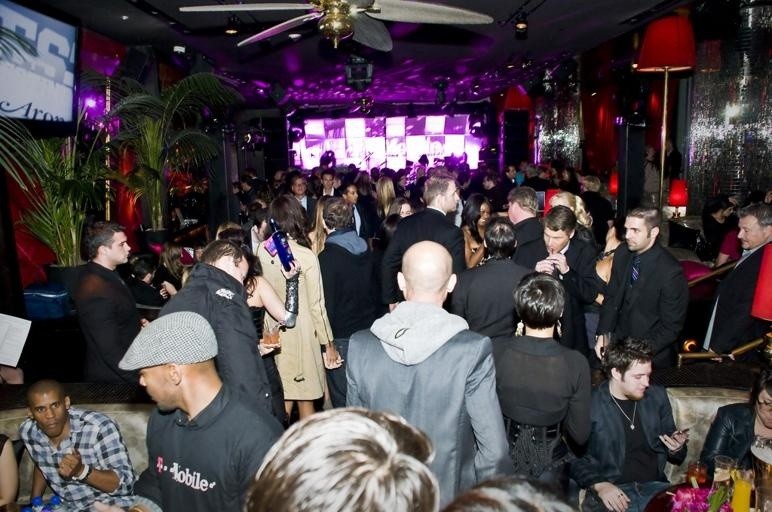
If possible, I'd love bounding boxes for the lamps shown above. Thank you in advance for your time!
[750,243,772,372]
[636,15,695,213]
[310,7,354,47]
[667,179,688,217]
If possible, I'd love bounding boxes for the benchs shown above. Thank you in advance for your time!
[5,382,148,407]
[0,403,156,512]
[579,386,749,512]
[136,301,161,321]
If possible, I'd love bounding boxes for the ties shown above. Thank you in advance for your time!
[630,257,640,284]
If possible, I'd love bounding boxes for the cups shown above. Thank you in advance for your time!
[728,468,752,511]
[685,458,708,486]
[261,328,281,346]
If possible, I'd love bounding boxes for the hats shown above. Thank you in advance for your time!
[117,310,218,373]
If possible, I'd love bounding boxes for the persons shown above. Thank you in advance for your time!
[0,141,772,512]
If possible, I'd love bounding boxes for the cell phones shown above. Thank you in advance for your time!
[665,428,690,441]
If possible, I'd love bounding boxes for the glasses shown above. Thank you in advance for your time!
[759,400,772,412]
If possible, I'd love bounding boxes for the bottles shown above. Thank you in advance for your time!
[711,455,736,501]
[22,494,67,512]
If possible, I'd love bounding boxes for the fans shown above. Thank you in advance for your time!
[179,0,496,55]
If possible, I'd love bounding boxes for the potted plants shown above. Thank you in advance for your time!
[0,80,159,291]
[75,57,242,253]
